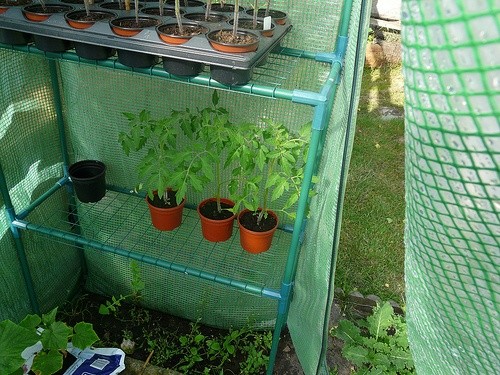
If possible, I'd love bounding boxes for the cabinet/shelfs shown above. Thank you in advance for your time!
[0,0,360,375]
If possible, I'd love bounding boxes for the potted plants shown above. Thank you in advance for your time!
[0,0,333,256]
